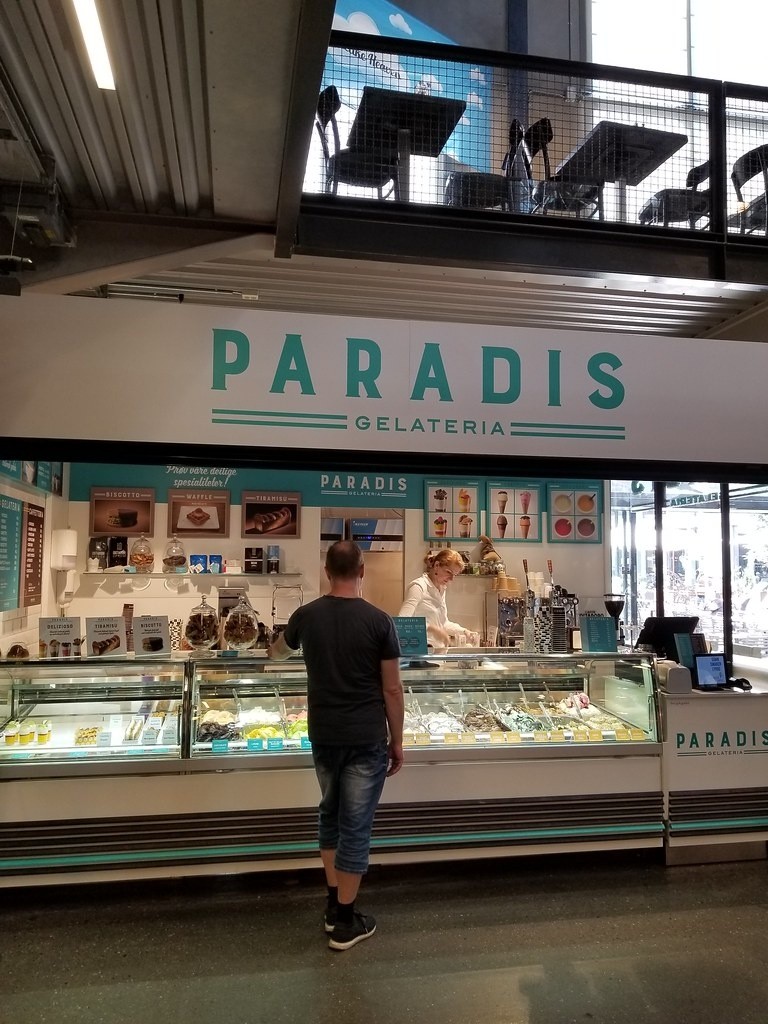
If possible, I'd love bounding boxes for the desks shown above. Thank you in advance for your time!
[556,121,688,223]
[345,86,467,202]
[727,192,765,230]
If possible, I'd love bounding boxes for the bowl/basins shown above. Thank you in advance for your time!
[554,494,573,512]
[576,494,595,514]
[553,518,573,538]
[576,517,596,538]
[522,605,568,652]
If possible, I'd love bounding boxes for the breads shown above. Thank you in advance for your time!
[187,508,210,523]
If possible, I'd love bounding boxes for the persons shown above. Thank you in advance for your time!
[399,549,478,656]
[266,540,404,948]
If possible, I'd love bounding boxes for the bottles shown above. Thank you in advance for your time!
[129,531,155,573]
[458,551,506,575]
[272,583,304,633]
[185,595,259,657]
[424,540,452,573]
[162,533,187,573]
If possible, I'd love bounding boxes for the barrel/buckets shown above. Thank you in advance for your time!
[189,554,223,573]
[266,545,280,573]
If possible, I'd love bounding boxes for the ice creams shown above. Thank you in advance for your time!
[520,516,532,539]
[520,491,531,514]
[497,515,508,538]
[497,490,508,513]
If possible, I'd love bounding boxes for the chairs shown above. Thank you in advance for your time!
[444,117,601,217]
[639,160,711,232]
[316,85,399,201]
[732,144,768,236]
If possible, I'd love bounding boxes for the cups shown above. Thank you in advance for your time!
[5,729,52,745]
[450,632,481,647]
[74,645,81,656]
[49,645,59,657]
[434,496,471,537]
[493,572,520,591]
[528,572,555,597]
[40,645,47,657]
[423,645,448,654]
[62,645,72,657]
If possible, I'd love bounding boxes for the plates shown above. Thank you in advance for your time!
[176,505,219,529]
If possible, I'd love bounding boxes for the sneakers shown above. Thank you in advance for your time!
[328,908,376,950]
[324,909,336,931]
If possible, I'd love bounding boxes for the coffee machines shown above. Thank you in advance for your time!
[602,593,625,645]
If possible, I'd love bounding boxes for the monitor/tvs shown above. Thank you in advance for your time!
[674,634,728,691]
[633,617,700,664]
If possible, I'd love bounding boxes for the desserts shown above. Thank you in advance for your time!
[75,726,102,745]
[255,507,291,533]
[106,508,138,527]
[4,719,53,744]
[554,495,595,537]
